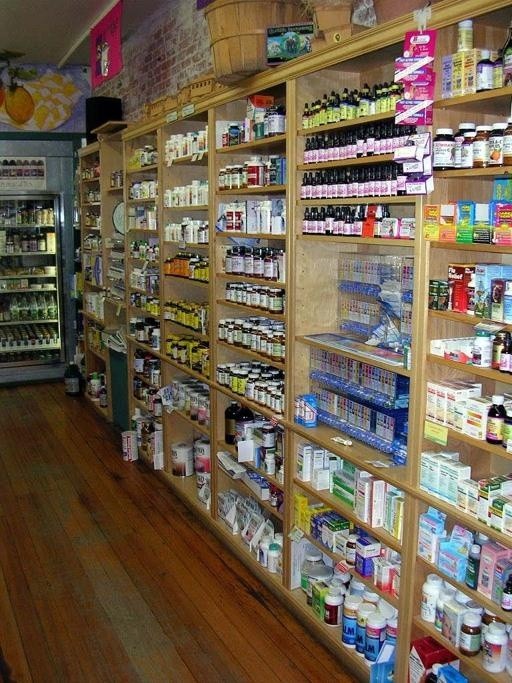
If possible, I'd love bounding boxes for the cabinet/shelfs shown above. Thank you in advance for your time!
[123,117,161,478]
[161,106,211,530]
[101,121,125,310]
[78,140,119,422]
[394,3,509,683]
[285,22,421,683]
[208,69,290,597]
[0,193,67,366]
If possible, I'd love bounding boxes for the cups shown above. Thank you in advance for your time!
[30,283,55,289]
[44,266,56,275]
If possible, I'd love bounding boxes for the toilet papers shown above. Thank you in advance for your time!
[195,438,212,473]
[197,471,212,502]
[170,442,195,478]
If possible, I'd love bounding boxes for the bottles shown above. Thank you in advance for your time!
[79,167,101,229]
[254,103,286,140]
[417,572,512,683]
[216,242,286,508]
[87,371,107,408]
[299,79,417,239]
[346,536,360,565]
[129,126,210,429]
[0,204,59,254]
[2,350,59,366]
[300,546,397,665]
[471,330,512,375]
[256,199,271,233]
[222,122,244,147]
[454,19,473,52]
[475,21,512,91]
[463,533,490,589]
[432,115,512,171]
[131,407,144,446]
[0,293,58,321]
[64,360,84,397]
[486,392,511,448]
[219,152,280,189]
[258,532,282,575]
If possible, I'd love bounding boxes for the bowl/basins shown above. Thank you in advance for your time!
[202,0,308,86]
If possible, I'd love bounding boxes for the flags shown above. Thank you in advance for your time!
[88,0,125,90]
